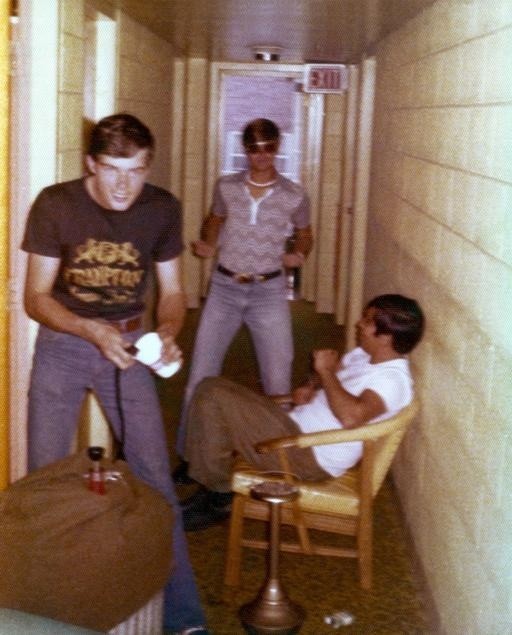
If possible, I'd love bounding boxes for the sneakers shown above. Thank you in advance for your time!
[171,459,198,486]
[180,486,235,532]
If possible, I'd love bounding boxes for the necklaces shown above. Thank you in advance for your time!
[244,169,278,188]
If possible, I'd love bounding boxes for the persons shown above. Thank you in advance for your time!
[18,113,211,635]
[179,294,426,537]
[167,117,314,487]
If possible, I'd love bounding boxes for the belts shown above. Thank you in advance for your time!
[216,264,282,285]
[111,316,142,335]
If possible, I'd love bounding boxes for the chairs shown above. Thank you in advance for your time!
[219,382,421,590]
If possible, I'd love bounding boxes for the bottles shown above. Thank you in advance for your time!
[85,444,108,498]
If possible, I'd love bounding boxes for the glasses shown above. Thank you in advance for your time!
[246,143,277,154]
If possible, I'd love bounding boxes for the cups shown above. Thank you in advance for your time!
[130,330,181,379]
